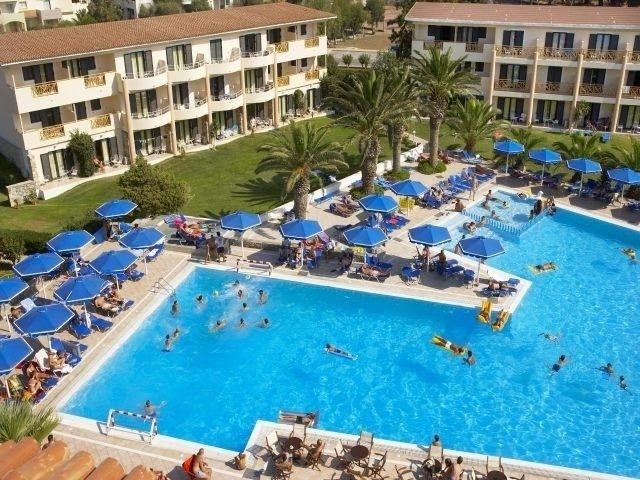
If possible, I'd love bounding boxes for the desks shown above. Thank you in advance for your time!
[350,444,368,462]
[485,470,505,480]
[421,459,444,475]
[285,437,302,455]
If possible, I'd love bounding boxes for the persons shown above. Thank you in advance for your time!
[358,266,390,278]
[429,186,444,201]
[343,196,360,208]
[468,222,477,232]
[462,351,475,366]
[586,116,597,131]
[282,236,335,268]
[479,307,490,323]
[599,362,615,375]
[42,434,53,450]
[430,336,464,355]
[280,413,315,428]
[8,220,138,395]
[614,246,635,253]
[93,155,103,170]
[455,199,465,212]
[160,223,270,353]
[491,210,497,218]
[537,333,561,346]
[137,400,157,418]
[433,249,446,263]
[192,435,463,480]
[326,343,348,356]
[483,190,492,209]
[469,175,479,201]
[618,376,628,389]
[545,355,568,379]
[419,246,430,261]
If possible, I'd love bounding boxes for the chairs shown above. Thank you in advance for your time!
[427,445,446,463]
[22,300,42,314]
[344,463,366,478]
[288,426,308,446]
[267,432,282,454]
[484,456,506,471]
[275,234,520,298]
[368,453,391,478]
[471,467,484,480]
[67,319,89,338]
[329,147,497,234]
[334,438,352,462]
[106,289,137,306]
[356,431,375,455]
[91,314,113,335]
[509,166,640,213]
[272,463,297,479]
[62,261,83,279]
[37,350,68,371]
[306,445,326,470]
[5,379,36,401]
[126,268,144,281]
[508,112,640,134]
[176,233,197,250]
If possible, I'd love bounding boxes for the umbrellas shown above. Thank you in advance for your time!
[219,180,506,283]
[493,139,640,208]
[0,199,166,399]
